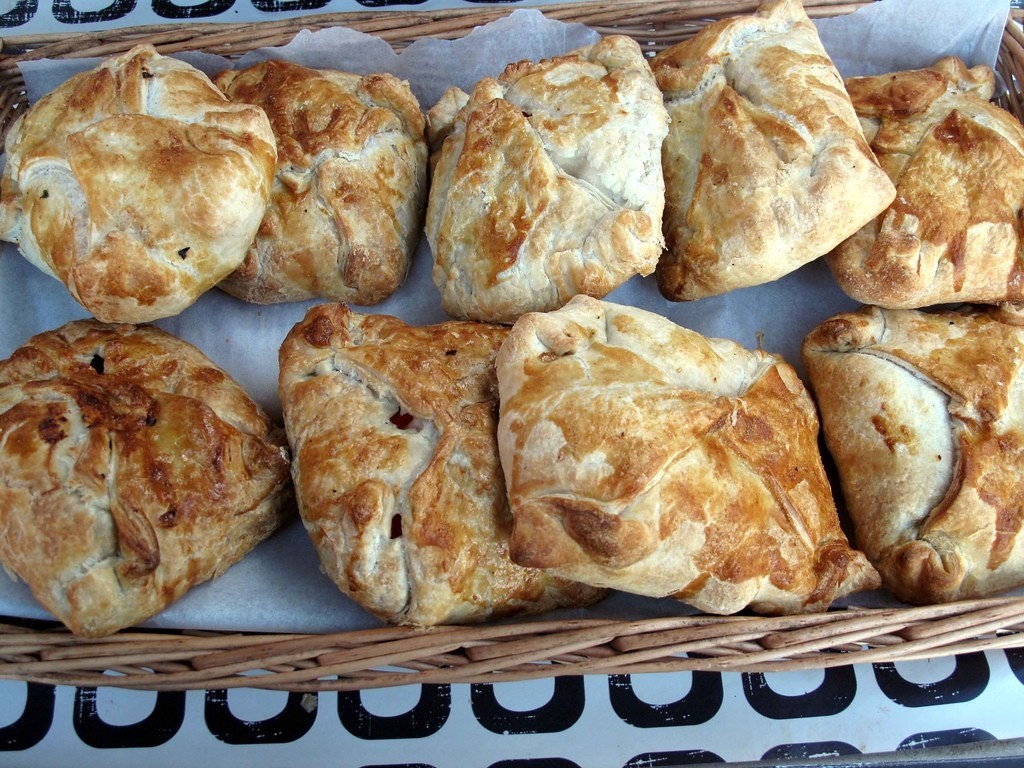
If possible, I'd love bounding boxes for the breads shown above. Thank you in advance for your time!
[1,0,1024,638]
